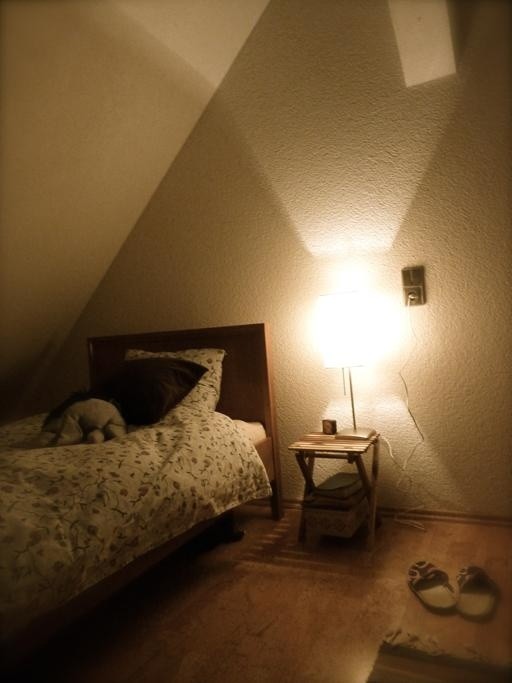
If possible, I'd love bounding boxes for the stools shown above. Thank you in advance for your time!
[288,431,380,552]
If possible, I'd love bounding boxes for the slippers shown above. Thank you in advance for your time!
[407,560,499,618]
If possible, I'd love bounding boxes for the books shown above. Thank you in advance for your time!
[315,471,363,499]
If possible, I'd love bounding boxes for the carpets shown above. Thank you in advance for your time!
[366,627,512,683]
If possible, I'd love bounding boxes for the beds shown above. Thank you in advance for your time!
[0,322,284,678]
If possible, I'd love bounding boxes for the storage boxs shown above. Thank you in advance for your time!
[303,496,369,538]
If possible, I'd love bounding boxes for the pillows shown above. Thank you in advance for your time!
[119,347,228,411]
[40,357,209,431]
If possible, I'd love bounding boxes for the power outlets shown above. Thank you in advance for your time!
[401,266,425,307]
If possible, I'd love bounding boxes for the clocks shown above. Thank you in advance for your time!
[322,419,336,434]
